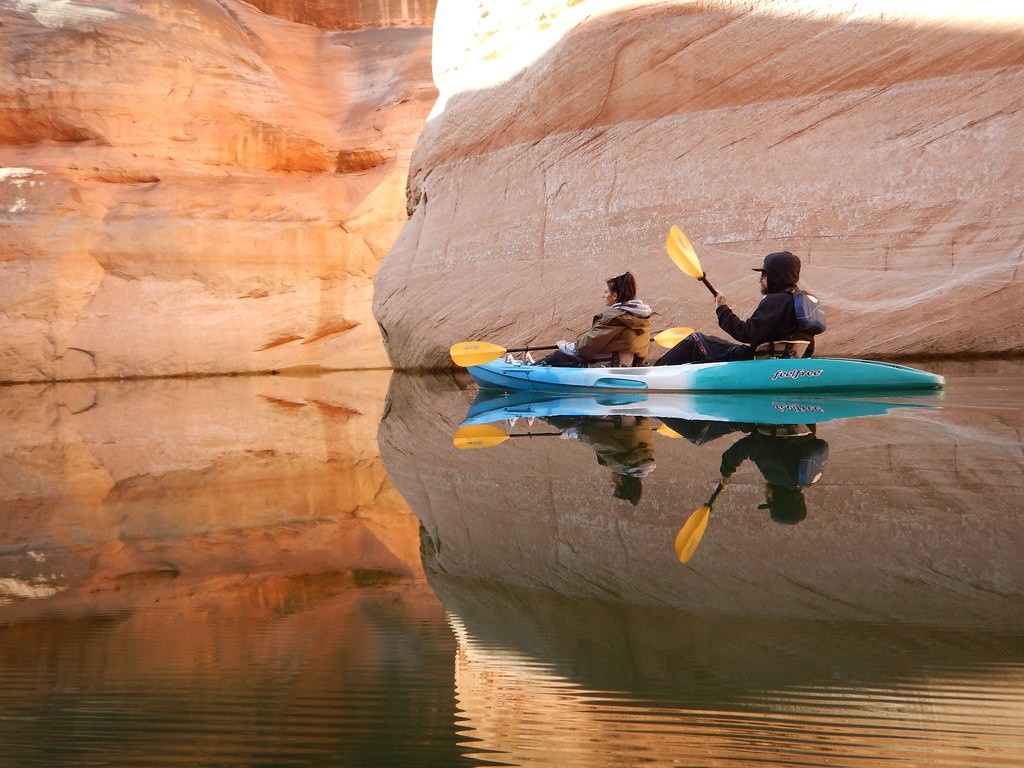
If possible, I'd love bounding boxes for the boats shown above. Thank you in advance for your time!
[466,341,948,389]
[457,389,945,437]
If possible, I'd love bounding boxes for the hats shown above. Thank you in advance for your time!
[752,268,764,271]
[758,504,770,510]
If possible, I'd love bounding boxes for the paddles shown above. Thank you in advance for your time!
[450,326,697,369]
[666,225,717,297]
[675,483,724,565]
[452,419,682,449]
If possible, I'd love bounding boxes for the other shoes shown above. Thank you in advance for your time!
[506,417,520,427]
[523,351,535,367]
[526,416,535,427]
[504,353,517,365]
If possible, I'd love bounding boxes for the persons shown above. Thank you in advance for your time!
[508,416,655,506]
[653,251,827,367]
[657,418,829,525]
[505,271,651,367]
